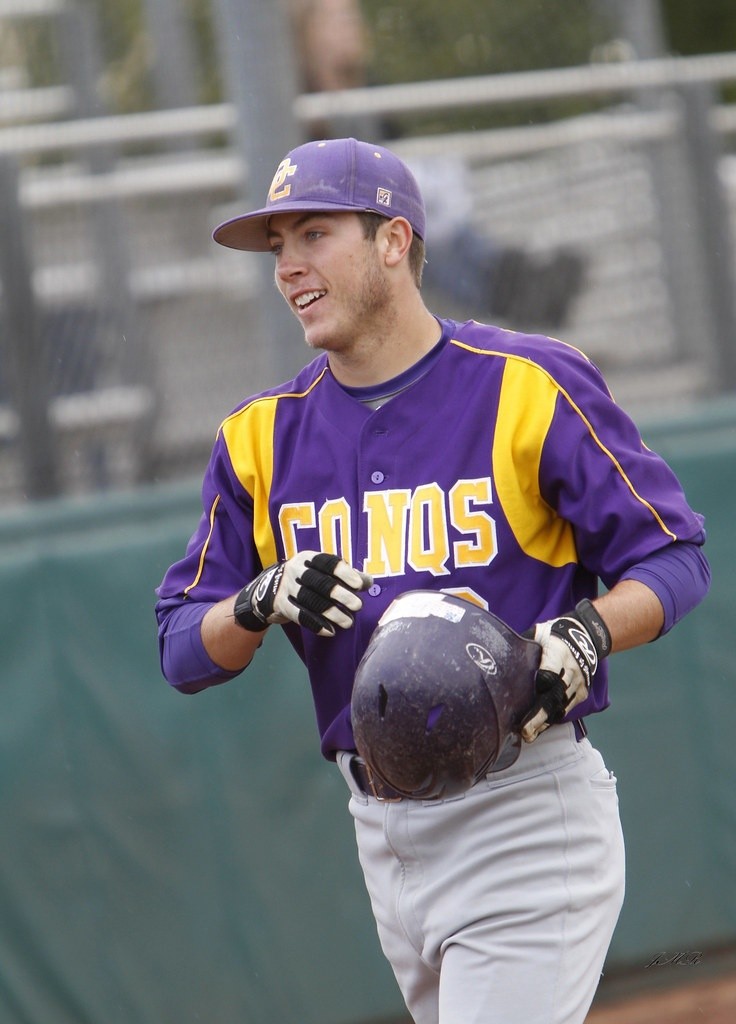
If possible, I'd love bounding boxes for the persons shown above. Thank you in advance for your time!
[158,135,710,1023]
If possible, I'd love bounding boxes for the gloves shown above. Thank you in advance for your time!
[512,600,616,749]
[231,559,368,643]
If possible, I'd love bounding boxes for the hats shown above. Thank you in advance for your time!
[212,151,431,249]
